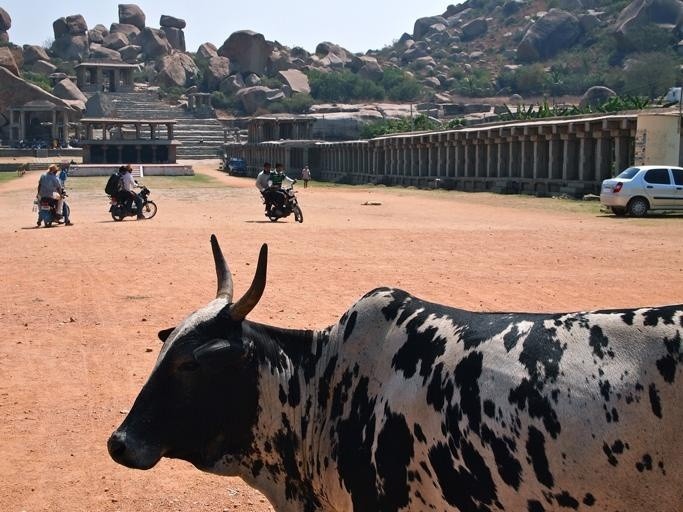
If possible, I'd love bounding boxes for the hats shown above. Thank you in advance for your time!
[48,164,60,173]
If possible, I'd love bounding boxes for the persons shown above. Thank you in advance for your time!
[111,166,134,214]
[255,162,275,215]
[36,175,74,227]
[267,164,298,216]
[302,165,311,188]
[36,164,66,221]
[123,164,146,220]
[58,169,66,187]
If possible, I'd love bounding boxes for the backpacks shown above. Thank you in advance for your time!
[104,172,122,195]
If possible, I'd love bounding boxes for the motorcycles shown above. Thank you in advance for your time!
[108,181,157,220]
[33,190,71,227]
[262,180,304,222]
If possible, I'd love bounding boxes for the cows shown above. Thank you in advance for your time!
[106,233,683,512]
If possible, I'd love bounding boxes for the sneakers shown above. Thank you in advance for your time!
[65,222,73,225]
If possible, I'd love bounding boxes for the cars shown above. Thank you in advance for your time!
[228,157,247,176]
[598,165,683,216]
